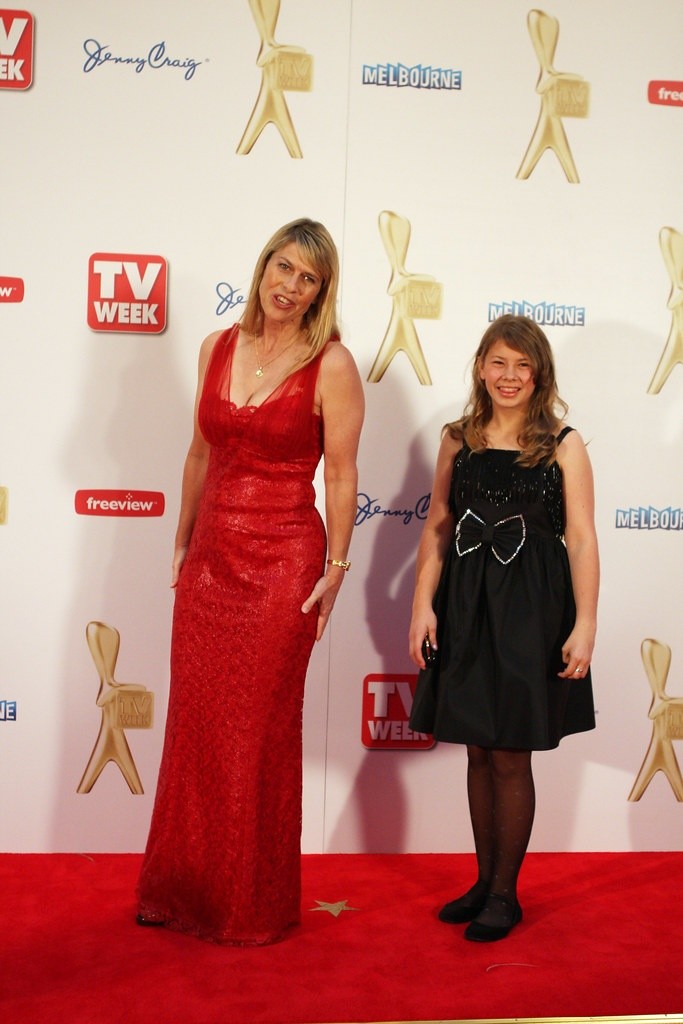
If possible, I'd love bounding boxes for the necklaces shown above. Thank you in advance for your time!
[253,332,298,378]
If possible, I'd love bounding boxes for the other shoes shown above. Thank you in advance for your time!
[134,913,165,926]
[439,884,484,923]
[465,891,522,943]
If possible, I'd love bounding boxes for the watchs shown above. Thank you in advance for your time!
[327,559,352,571]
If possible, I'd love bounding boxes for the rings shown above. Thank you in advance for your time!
[575,667,583,674]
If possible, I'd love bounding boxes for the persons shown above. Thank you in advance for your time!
[409,315,598,941]
[135,218,365,947]
